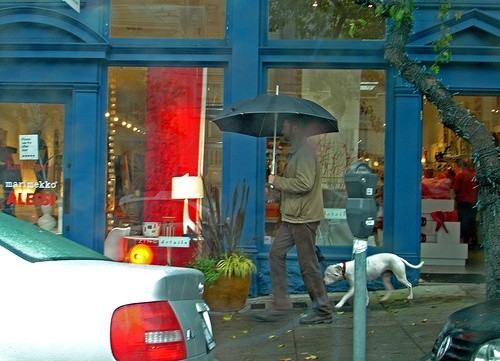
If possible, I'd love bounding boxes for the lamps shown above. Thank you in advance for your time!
[171,176,204,235]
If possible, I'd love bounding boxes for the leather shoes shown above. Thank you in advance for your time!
[252,311,292,323]
[298,315,333,327]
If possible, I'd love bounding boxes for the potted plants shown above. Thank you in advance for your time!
[182,177,257,312]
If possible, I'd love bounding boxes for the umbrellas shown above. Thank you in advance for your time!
[210,84,339,188]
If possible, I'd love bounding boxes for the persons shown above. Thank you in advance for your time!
[450,158,481,249]
[254,115,332,324]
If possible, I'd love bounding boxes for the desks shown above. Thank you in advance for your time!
[123,235,205,266]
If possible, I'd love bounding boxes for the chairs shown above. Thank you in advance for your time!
[104,226,131,261]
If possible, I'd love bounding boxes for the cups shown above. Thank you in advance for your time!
[162,216,176,238]
[142,221,161,238]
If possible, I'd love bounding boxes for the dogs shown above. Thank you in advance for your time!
[322,251,425,308]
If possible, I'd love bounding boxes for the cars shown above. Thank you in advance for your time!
[423,298,500,361]
[0,210,217,361]
[119,189,212,220]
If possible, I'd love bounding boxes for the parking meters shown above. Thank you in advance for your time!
[344,161,379,360]
